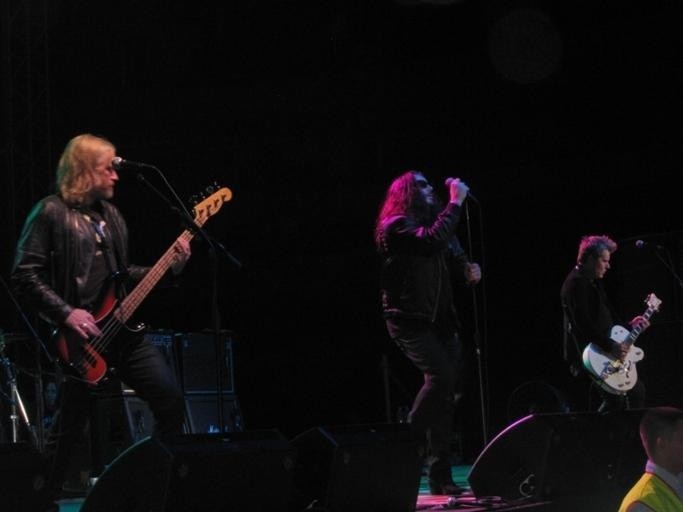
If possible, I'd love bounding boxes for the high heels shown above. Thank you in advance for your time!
[426,477,468,495]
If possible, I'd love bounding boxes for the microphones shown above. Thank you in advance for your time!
[635,239,661,251]
[110,156,157,174]
[445,178,479,205]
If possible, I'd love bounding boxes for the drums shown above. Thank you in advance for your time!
[507,379,571,435]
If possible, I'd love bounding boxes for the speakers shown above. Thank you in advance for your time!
[79,434,265,512]
[468,409,667,500]
[1,441,54,511]
[268,423,421,511]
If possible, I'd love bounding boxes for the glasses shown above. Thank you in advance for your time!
[412,179,431,189]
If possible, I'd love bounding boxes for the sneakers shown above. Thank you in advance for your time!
[62,470,100,492]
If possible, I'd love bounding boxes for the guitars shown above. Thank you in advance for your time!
[49,179,232,395]
[582,293,663,396]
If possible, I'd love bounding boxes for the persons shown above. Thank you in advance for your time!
[618,406,683,512]
[560,234,650,413]
[9,133,190,472]
[31,380,60,428]
[374,169,482,496]
